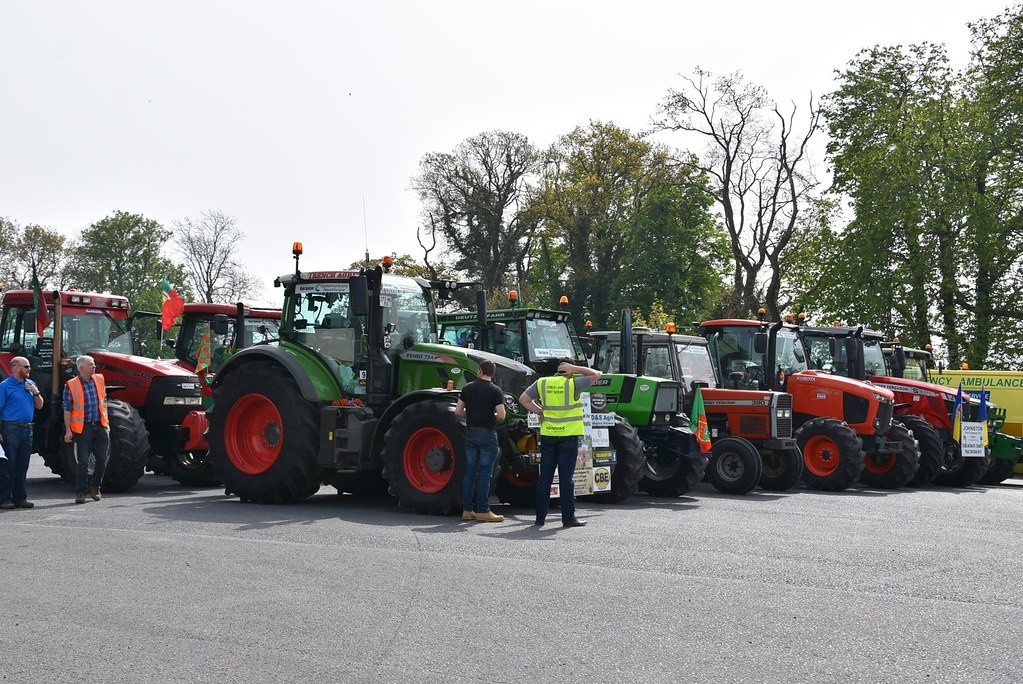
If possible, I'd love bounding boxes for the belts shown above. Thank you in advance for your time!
[471,427,493,432]
[1,420,33,427]
[84,421,101,425]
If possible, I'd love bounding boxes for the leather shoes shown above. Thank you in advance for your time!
[535,518,545,525]
[563,520,586,527]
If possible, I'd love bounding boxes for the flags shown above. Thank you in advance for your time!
[33,262,51,339]
[689,387,713,454]
[194,324,211,374]
[978,389,988,446]
[951,385,961,444]
[162,278,184,332]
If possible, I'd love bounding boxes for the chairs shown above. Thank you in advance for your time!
[33,337,67,367]
[321,313,352,342]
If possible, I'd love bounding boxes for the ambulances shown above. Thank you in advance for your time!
[841,345,1023,486]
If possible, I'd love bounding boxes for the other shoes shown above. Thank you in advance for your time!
[12,499,34,508]
[0,498,15,509]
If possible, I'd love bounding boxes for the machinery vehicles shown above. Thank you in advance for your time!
[582,318,804,495]
[693,313,921,494]
[331,308,607,360]
[784,314,1008,489]
[203,240,552,518]
[408,290,710,505]
[158,293,311,418]
[0,288,224,495]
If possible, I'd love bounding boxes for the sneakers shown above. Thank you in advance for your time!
[75,490,86,502]
[475,510,504,521]
[462,511,477,519]
[89,485,101,500]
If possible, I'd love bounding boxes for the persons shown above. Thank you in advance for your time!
[0,356,44,509]
[455,360,506,522]
[519,357,601,527]
[62,355,112,504]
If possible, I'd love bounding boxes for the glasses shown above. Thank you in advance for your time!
[13,365,32,369]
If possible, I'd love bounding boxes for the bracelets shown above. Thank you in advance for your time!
[33,392,40,396]
